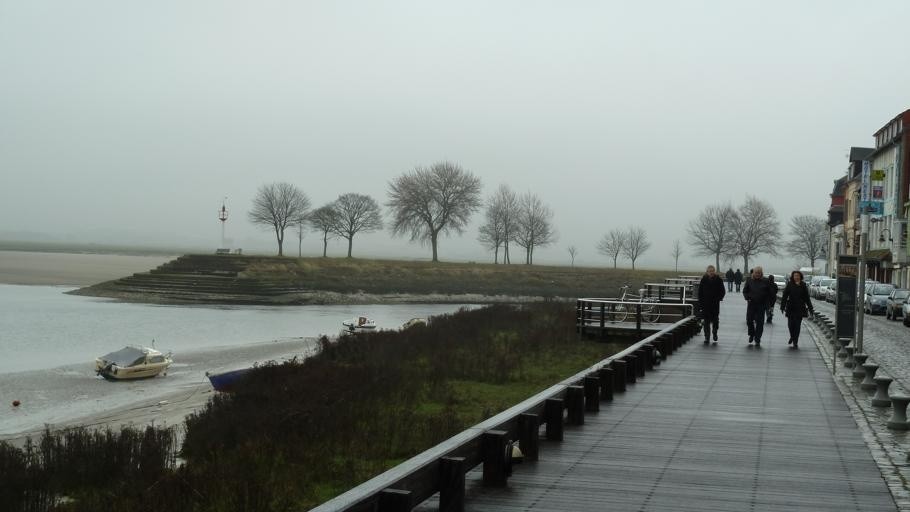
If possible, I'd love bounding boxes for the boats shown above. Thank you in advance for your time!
[399,317,427,331]
[343,316,377,328]
[94,343,174,381]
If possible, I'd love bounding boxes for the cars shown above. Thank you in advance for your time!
[802,275,910,326]
[773,275,786,290]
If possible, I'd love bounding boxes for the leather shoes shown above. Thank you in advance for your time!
[755,336,760,342]
[789,337,793,345]
[749,335,753,342]
[794,342,799,350]
[713,334,717,341]
[705,338,709,343]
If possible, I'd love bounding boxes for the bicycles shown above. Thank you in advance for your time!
[608,282,661,323]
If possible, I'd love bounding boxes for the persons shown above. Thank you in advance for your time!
[698,265,726,344]
[779,270,814,347]
[742,265,772,345]
[733,269,744,294]
[746,268,754,282]
[725,267,735,293]
[765,273,780,324]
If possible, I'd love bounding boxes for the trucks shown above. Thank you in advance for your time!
[800,266,822,275]
